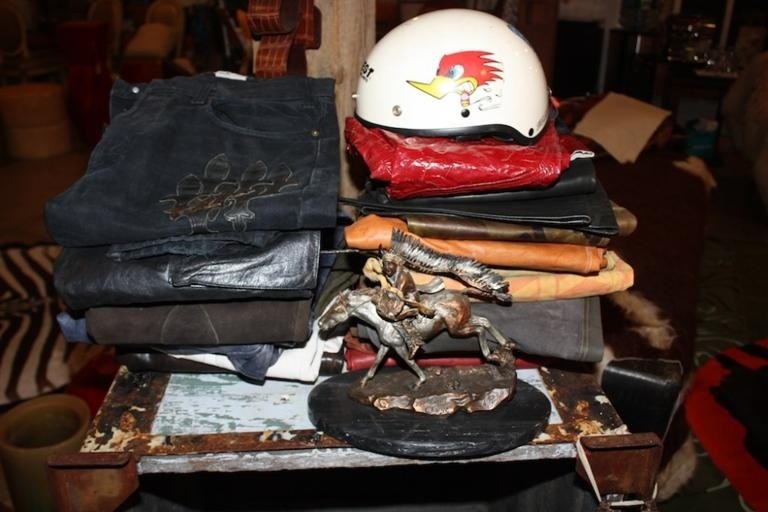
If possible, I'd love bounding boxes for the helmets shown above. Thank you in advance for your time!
[352,8,551,145]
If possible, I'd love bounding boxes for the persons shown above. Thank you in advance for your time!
[383,255,421,320]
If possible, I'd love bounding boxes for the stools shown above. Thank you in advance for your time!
[0,83,71,159]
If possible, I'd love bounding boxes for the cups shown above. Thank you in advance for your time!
[0,393,91,512]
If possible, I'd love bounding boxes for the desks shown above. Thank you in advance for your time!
[79,362,630,475]
